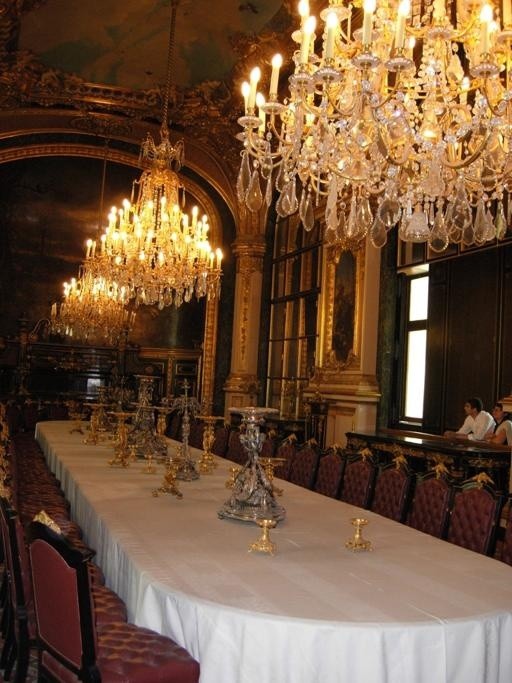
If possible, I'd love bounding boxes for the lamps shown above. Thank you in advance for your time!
[233,0,512,255]
[84,0,226,312]
[51,138,136,345]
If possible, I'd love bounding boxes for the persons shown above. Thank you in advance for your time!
[443,396,495,442]
[483,393,512,446]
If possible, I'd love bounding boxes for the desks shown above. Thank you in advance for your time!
[35,415,510,683]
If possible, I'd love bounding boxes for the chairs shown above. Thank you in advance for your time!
[0,401,201,682]
[121,410,509,566]
